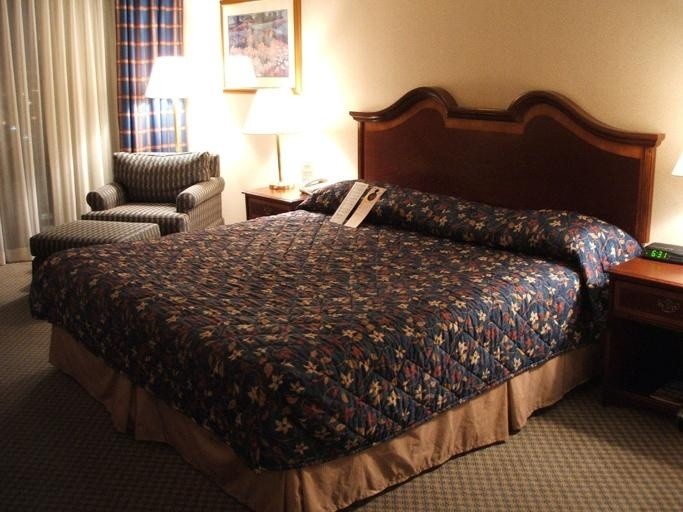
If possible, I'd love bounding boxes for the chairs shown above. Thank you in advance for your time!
[81,150,226,236]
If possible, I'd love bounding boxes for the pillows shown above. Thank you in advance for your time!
[303,177,642,327]
[113,152,211,202]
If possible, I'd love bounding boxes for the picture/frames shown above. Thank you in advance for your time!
[218,0,303,96]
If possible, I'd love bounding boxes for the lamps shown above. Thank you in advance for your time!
[242,89,298,191]
[144,56,199,153]
[671,141,683,176]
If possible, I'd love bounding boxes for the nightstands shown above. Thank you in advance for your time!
[599,246,683,425]
[240,179,332,221]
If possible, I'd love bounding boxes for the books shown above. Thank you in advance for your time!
[647,376,681,407]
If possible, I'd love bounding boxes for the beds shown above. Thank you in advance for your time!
[29,85,664,511]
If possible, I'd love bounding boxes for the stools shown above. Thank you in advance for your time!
[27,221,162,319]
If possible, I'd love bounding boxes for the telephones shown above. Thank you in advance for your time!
[299,178,332,196]
